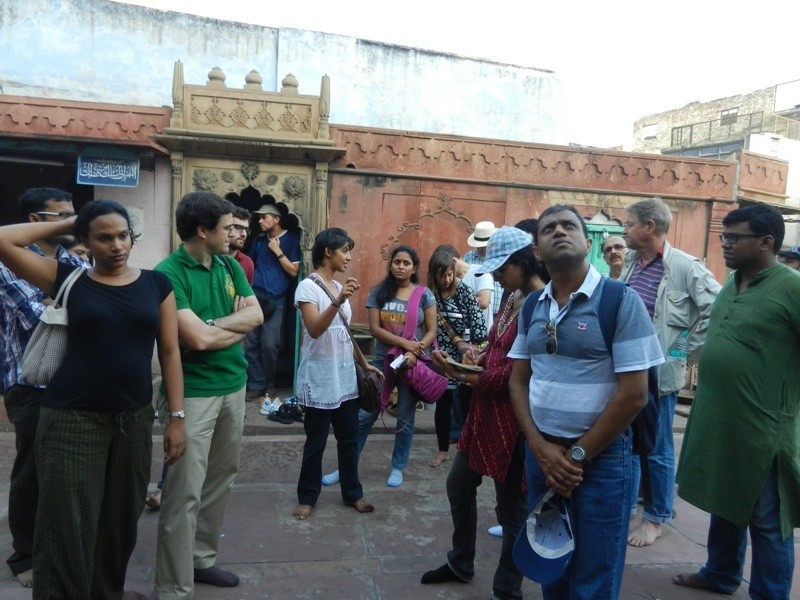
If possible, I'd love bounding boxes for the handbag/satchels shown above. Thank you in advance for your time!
[251,286,275,319]
[458,344,482,386]
[354,362,380,412]
[22,267,87,385]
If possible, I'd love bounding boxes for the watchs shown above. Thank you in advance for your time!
[169,410,185,419]
[277,251,285,258]
[460,371,467,384]
[568,442,590,464]
[205,319,215,326]
[331,299,340,307]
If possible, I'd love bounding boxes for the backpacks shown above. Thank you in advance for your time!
[380,286,448,411]
[523,279,660,454]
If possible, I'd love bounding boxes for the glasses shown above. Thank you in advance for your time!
[233,224,251,235]
[603,244,628,252]
[218,224,231,232]
[36,211,76,219]
[718,232,761,244]
[490,261,515,276]
[545,318,558,357]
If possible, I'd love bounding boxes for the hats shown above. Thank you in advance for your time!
[467,221,500,248]
[777,247,800,259]
[510,489,575,583]
[473,225,533,277]
[252,204,281,216]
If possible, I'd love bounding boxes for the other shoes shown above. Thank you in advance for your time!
[279,403,304,422]
[267,411,292,426]
[283,397,296,405]
[259,397,283,415]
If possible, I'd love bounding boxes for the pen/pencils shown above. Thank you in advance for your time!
[413,334,425,355]
[469,341,477,367]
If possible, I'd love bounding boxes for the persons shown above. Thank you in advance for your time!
[775,247,800,271]
[620,197,724,548]
[601,237,631,280]
[0,188,186,600]
[671,202,800,600]
[419,217,551,600]
[321,245,437,486]
[152,191,265,600]
[227,203,300,414]
[295,226,385,521]
[505,205,667,600]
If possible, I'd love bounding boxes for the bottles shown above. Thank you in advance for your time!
[671,330,688,358]
